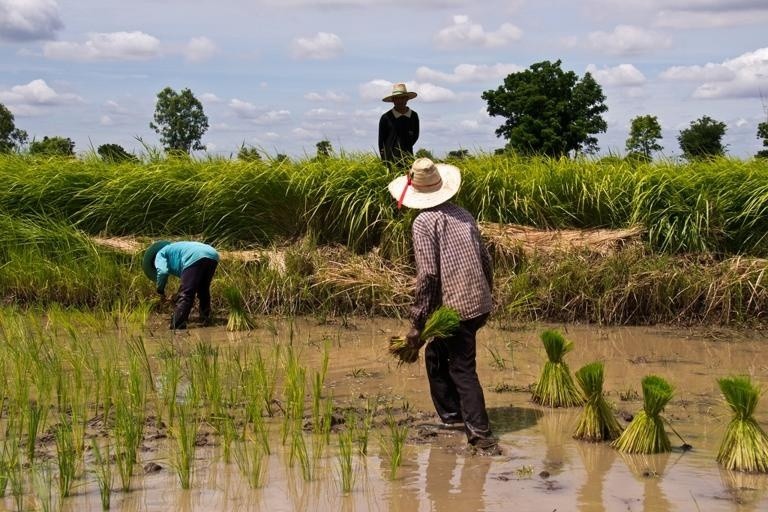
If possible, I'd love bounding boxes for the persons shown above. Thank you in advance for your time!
[388,157,502,449]
[142,240,220,328]
[378,84,420,168]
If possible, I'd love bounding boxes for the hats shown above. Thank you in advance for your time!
[142,241,171,281]
[382,83,417,102]
[388,158,462,210]
